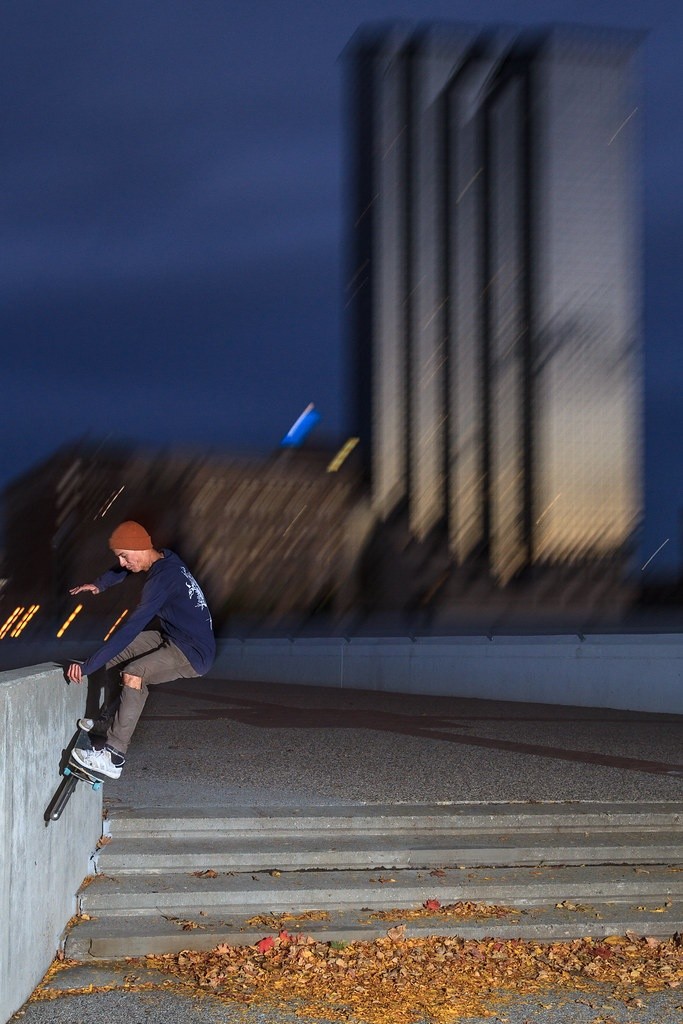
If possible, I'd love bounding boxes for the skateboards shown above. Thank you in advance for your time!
[63,727,107,791]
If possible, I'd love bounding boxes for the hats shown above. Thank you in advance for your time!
[109,521,153,550]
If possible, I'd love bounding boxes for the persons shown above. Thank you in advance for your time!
[65,519,216,779]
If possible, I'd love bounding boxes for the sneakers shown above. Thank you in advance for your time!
[79,717,108,738]
[71,746,125,779]
